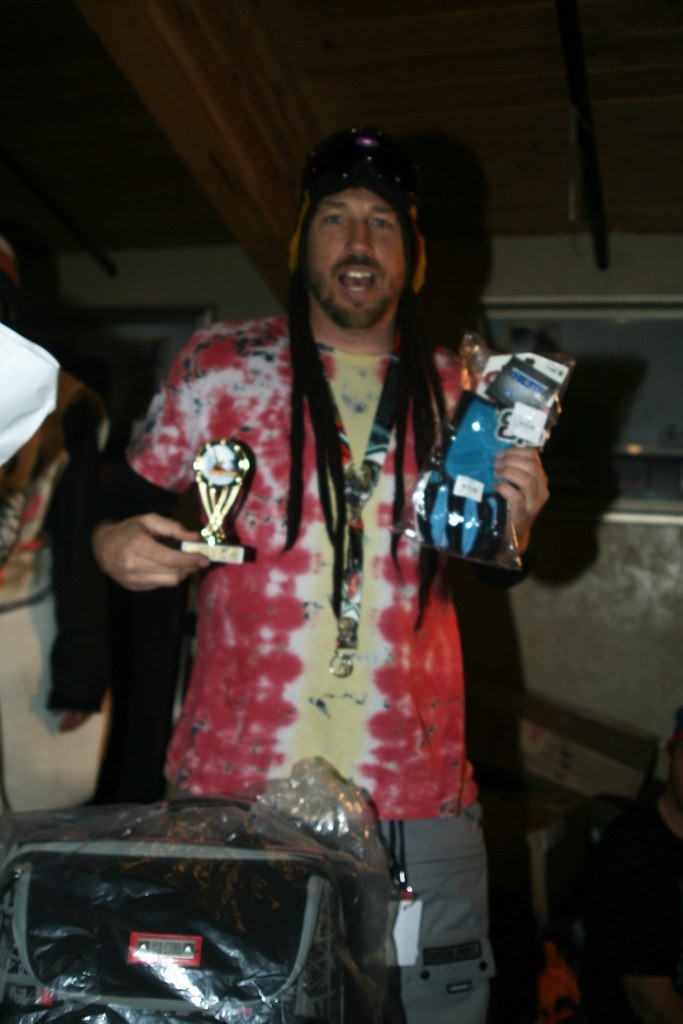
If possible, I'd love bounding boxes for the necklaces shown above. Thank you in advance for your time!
[300,320,402,677]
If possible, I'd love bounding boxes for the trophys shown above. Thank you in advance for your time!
[178,434,255,564]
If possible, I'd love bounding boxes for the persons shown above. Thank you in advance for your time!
[0,241,129,827]
[587,706,683,1024]
[83,106,553,1024]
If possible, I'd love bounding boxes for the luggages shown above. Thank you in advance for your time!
[0,797,363,1024]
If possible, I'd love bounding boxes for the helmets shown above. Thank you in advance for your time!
[310,126,413,195]
